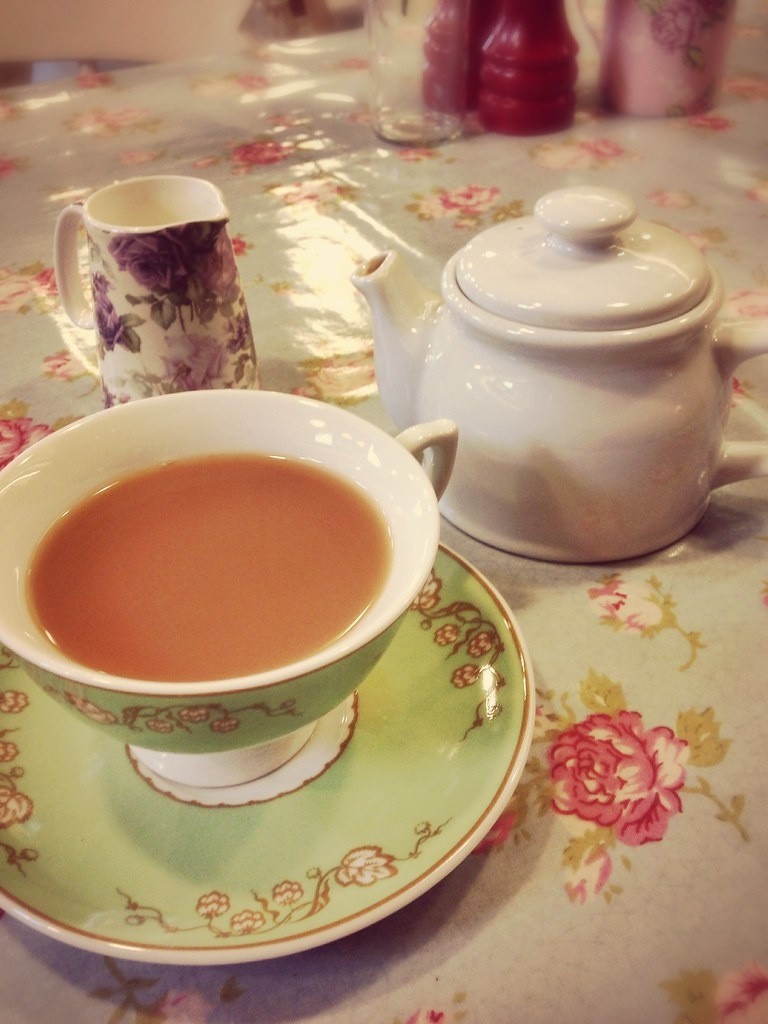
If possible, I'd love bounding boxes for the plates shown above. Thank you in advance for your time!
[0,541,536,970]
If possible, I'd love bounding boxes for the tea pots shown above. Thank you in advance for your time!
[350,188,768,561]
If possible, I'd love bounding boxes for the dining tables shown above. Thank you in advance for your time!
[0,0,768,1024]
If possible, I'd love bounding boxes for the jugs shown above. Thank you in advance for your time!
[53,175,260,413]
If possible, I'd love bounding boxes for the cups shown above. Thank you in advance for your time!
[575,0,742,115]
[0,390,455,785]
[364,0,470,145]
[421,0,482,111]
[478,0,578,136]
